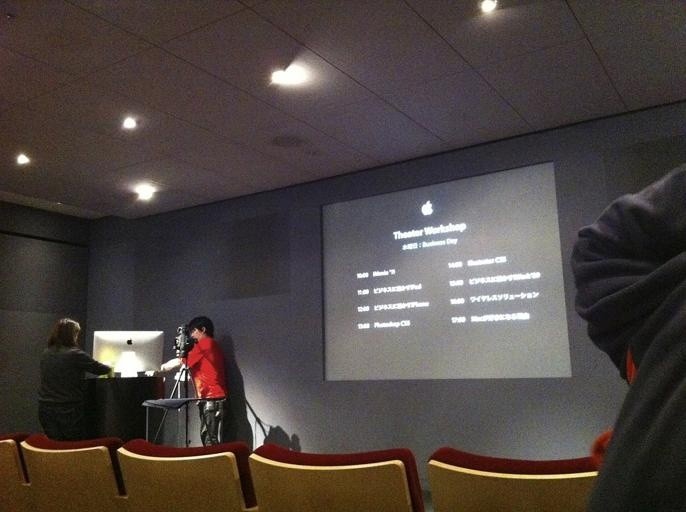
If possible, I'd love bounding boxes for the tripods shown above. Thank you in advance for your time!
[151,366,215,447]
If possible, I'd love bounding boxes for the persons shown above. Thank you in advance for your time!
[571,162,686,512]
[38,319,113,442]
[144,316,231,449]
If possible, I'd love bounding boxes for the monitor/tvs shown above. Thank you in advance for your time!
[92,330,165,379]
